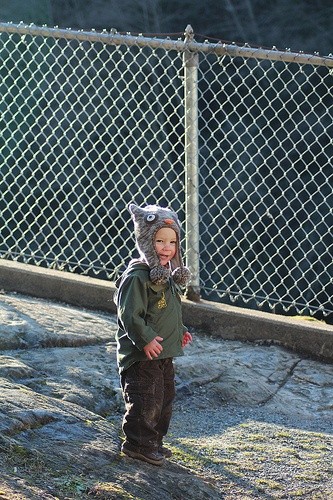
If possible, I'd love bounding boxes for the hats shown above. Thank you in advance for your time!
[129,204,191,284]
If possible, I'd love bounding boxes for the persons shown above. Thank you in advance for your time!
[113,204,192,466]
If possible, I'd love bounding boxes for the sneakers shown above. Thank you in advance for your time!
[120,441,172,466]
